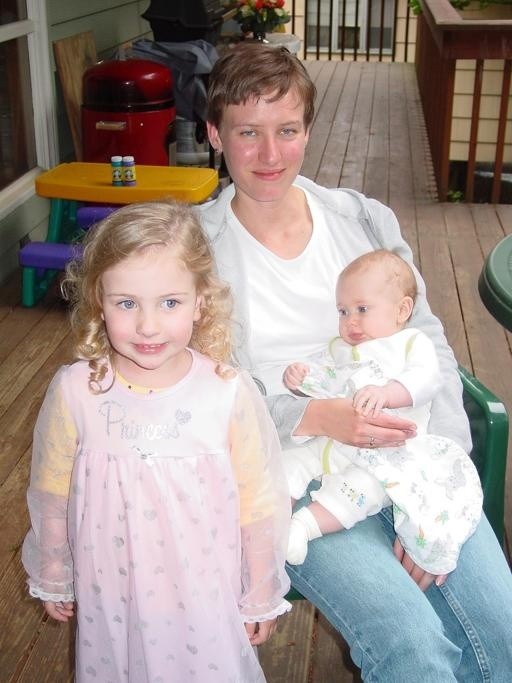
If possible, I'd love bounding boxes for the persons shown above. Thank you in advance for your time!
[21,198,293,682]
[180,42,512,682]
[281,249,484,575]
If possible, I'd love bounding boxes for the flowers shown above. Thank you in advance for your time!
[219,0,293,33]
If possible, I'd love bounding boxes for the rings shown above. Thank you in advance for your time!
[371,438,374,445]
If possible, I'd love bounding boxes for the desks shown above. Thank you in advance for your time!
[18,161,219,308]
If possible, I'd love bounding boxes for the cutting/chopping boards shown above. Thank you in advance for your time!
[52,30,97,162]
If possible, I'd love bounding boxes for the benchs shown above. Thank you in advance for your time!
[19,206,124,308]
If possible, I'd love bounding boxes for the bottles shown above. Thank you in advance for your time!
[110,156,137,188]
